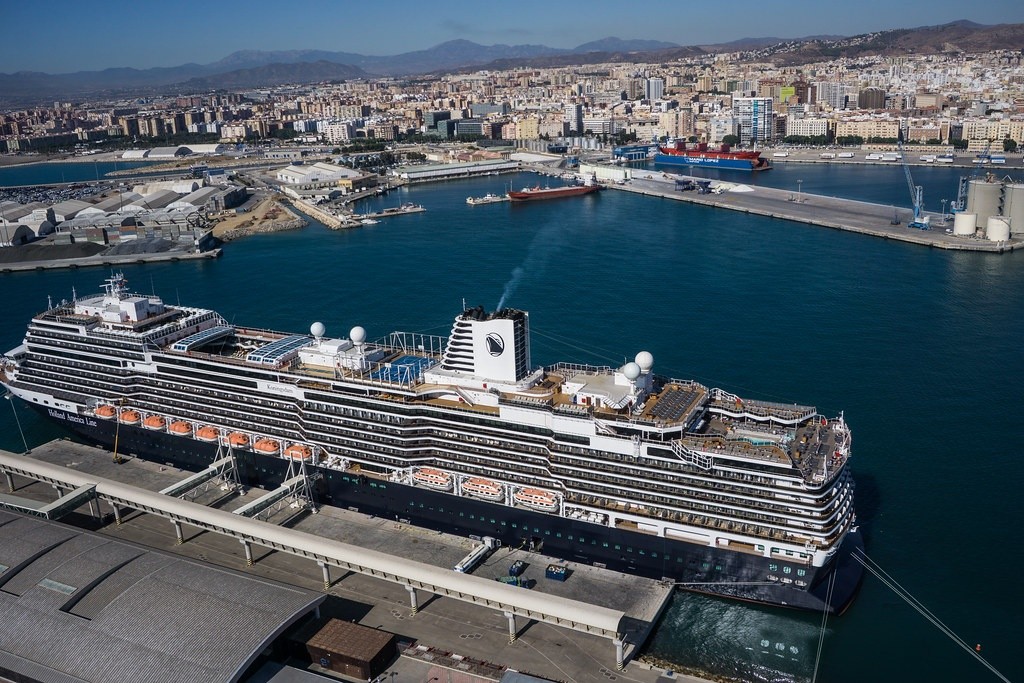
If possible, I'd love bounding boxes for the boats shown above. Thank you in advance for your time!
[143,415,166,431]
[223,432,250,449]
[461,477,505,502]
[194,426,220,442]
[254,437,279,455]
[94,406,118,419]
[507,183,603,202]
[167,419,193,436]
[284,444,312,461]
[119,410,141,424]
[652,138,773,172]
[413,468,453,492]
[514,487,559,513]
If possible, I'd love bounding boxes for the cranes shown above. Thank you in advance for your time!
[895,139,931,231]
[946,138,998,221]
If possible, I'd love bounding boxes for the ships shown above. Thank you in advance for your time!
[0,267,868,620]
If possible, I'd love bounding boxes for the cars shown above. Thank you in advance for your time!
[0,184,113,204]
